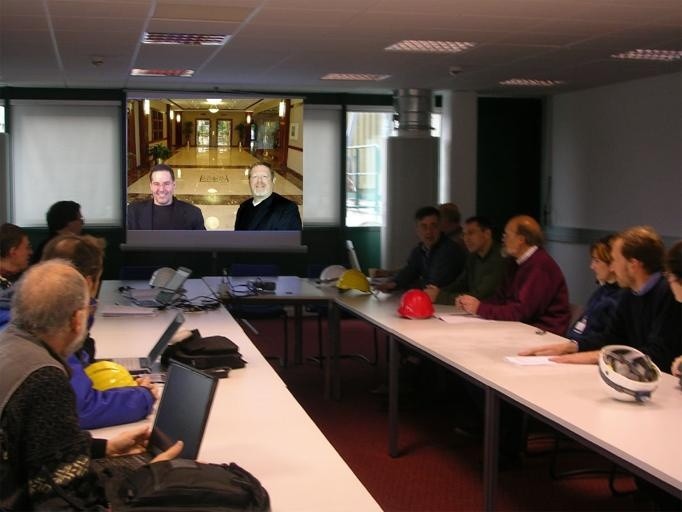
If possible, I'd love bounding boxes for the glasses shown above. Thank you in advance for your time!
[664,270,674,278]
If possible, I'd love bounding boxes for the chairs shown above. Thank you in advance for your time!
[226,264,288,367]
[112,265,175,280]
[303,264,379,369]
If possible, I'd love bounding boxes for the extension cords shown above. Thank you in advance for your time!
[220,284,230,295]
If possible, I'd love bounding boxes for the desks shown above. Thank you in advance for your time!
[91,275,382,512]
[302,273,682,510]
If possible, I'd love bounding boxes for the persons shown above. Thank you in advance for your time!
[235,162,302,231]
[128,164,206,231]
[372,203,571,373]
[523,225,681,393]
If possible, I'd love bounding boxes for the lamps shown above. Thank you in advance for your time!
[207,102,221,114]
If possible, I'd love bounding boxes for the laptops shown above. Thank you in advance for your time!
[130,265,193,308]
[91,357,218,473]
[94,312,186,376]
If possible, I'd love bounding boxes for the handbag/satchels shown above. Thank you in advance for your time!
[162,337,248,371]
[41,458,269,512]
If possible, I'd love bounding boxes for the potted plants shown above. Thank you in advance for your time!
[147,143,172,165]
[182,120,193,147]
[234,123,245,151]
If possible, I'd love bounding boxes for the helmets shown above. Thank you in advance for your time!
[84,360,138,392]
[599,345,662,401]
[321,264,347,287]
[149,268,175,288]
[337,270,369,293]
[397,289,435,318]
